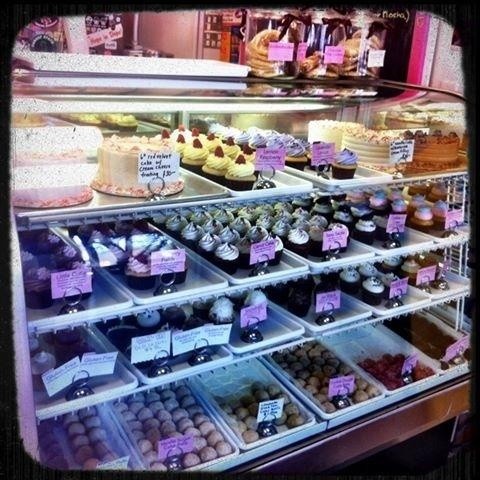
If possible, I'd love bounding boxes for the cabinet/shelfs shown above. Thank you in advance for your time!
[10,94,472,476]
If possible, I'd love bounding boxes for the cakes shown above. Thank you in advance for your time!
[11,102,186,209]
[246,28,287,77]
[305,103,465,175]
[331,39,360,75]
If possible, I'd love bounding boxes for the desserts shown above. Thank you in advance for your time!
[11,175,466,471]
[156,121,360,194]
